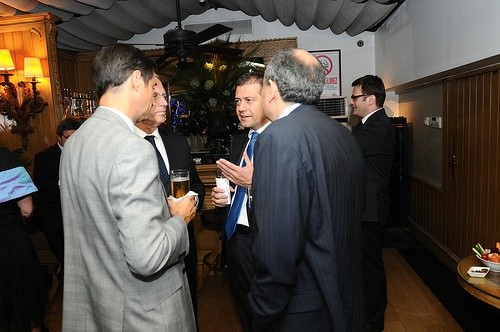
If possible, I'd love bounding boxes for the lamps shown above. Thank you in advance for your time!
[22,56,48,112]
[0,49,17,97]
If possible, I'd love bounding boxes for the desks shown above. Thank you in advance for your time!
[457,252,500,309]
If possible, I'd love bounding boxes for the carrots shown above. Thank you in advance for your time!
[490,254,500,263]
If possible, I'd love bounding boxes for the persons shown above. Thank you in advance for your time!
[210,71,272,332]
[240,49,368,332]
[352,74,396,332]
[0,148,49,332]
[59,43,199,332]
[137,73,205,332]
[32,118,80,237]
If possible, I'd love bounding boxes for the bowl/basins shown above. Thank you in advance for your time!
[476,253,500,272]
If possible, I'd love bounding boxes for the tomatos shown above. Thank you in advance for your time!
[482,254,488,259]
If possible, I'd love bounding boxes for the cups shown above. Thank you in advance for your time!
[170,169,190,199]
[215,177,231,204]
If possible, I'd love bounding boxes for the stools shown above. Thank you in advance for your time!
[196,209,237,296]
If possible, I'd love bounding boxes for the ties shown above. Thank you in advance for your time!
[225,132,259,240]
[145,136,172,198]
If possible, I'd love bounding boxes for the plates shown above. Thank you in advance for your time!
[467,266,490,278]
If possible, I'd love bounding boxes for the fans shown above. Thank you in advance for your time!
[108,0,246,66]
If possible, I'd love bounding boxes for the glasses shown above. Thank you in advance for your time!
[351,94,369,99]
[63,135,70,139]
